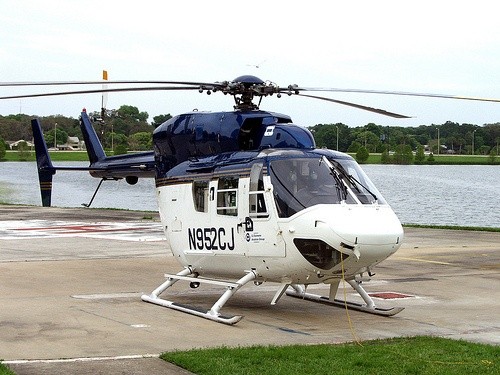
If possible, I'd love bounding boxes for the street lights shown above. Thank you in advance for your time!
[436,127,441,154]
[54,122,58,148]
[472,130,477,155]
[335,125,340,151]
[111,124,114,151]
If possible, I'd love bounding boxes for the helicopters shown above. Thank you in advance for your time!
[0,73,500,325]
